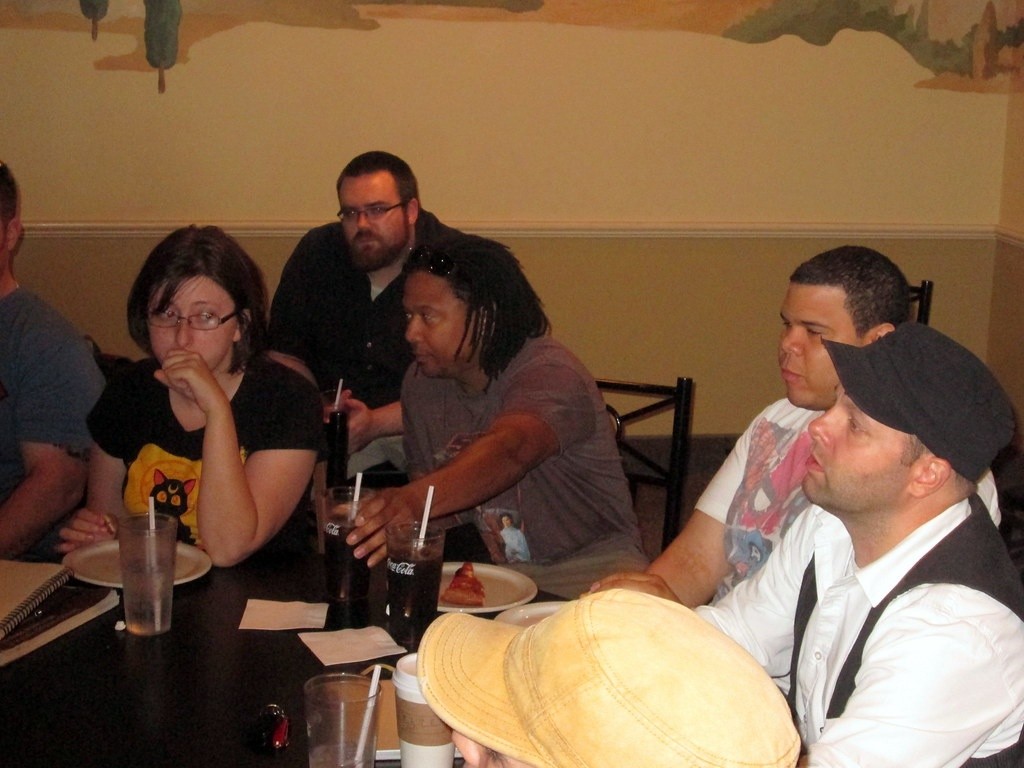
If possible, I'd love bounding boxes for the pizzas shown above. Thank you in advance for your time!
[440,561,487,607]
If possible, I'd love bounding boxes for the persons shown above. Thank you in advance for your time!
[264,151,468,491]
[0,161,105,565]
[587,244,1001,608]
[415,587,802,768]
[497,511,531,564]
[585,324,1023,768]
[328,232,651,601]
[52,224,324,568]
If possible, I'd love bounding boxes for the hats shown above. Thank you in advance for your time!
[821,322,1016,481]
[416,587,802,768]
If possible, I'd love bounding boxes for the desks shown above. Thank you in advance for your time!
[0,512,572,768]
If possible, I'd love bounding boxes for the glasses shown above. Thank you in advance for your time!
[144,310,240,330]
[407,244,474,285]
[337,199,408,224]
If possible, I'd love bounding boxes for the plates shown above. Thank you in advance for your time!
[63,539,211,588]
[436,562,537,612]
[494,602,570,627]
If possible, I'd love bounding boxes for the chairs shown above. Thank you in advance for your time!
[593,378,693,559]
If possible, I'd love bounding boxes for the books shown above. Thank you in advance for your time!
[0,557,121,665]
[329,677,465,761]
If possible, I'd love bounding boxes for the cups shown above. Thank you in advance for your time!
[391,653,454,768]
[304,672,381,768]
[320,389,352,418]
[384,521,446,644]
[323,488,375,602]
[117,514,177,635]
